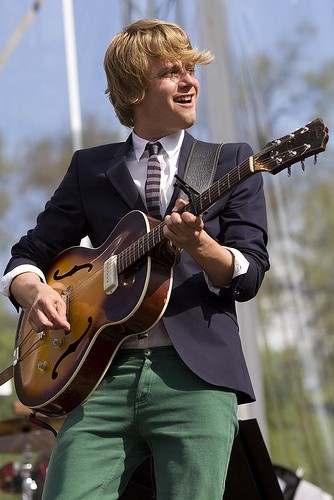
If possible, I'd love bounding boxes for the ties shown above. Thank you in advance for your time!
[145,142,163,221]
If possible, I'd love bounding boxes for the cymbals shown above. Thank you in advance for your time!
[0,417,63,455]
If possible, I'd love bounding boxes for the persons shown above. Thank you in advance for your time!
[0,17,270,500]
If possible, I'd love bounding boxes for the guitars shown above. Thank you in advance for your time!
[10,116,331,420]
